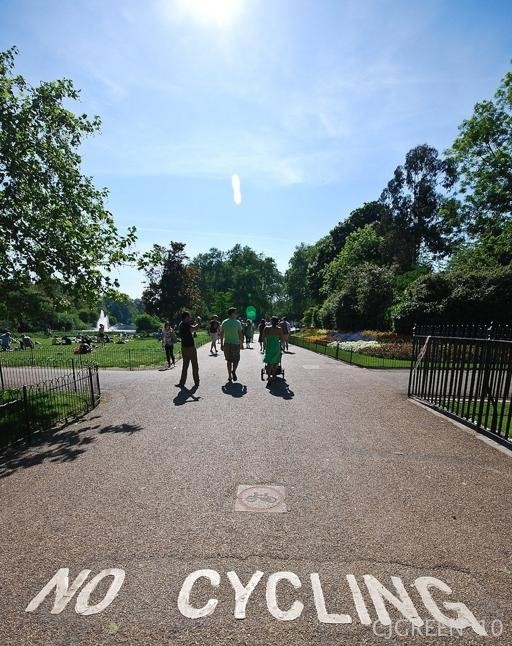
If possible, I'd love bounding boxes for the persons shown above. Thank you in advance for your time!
[158,320,178,370]
[44,314,291,356]
[261,315,286,382]
[219,306,244,382]
[175,310,201,388]
[18,334,35,352]
[0,331,21,352]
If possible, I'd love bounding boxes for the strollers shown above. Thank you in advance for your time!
[261,345,286,382]
